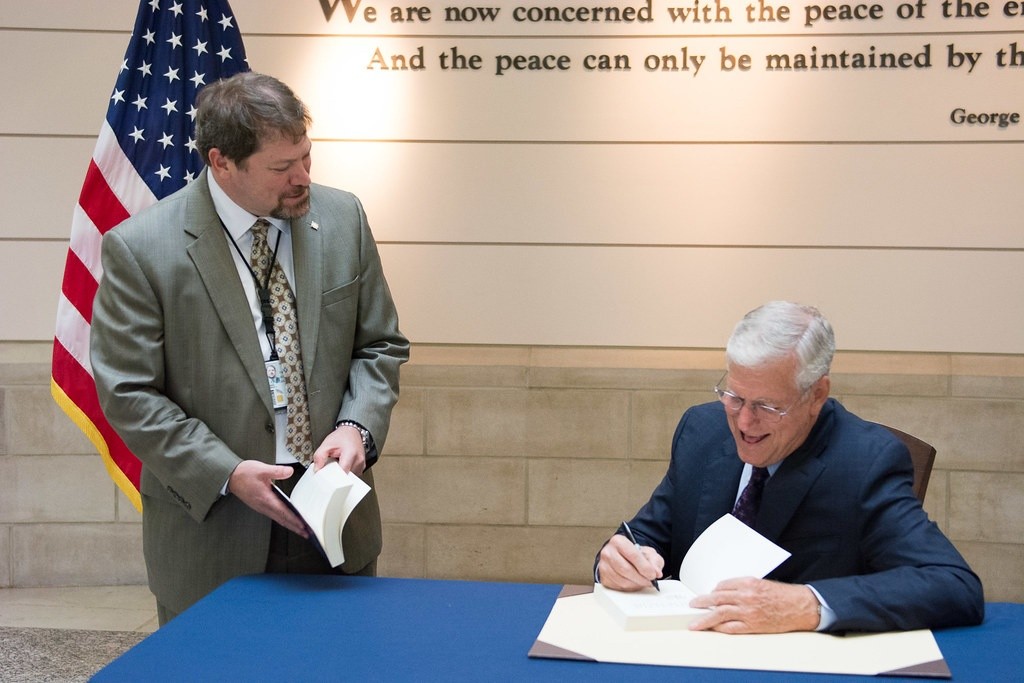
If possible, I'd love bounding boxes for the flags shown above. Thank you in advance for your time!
[48,0,253,516]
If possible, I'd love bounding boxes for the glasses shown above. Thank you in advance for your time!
[714,369,822,424]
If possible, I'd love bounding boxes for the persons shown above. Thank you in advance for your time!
[88,72,413,633]
[593,298,984,634]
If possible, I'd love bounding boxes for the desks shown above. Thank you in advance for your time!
[84,572,1024,683]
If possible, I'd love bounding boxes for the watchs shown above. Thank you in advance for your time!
[338,421,374,452]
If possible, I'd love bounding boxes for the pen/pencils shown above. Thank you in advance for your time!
[622,521,661,593]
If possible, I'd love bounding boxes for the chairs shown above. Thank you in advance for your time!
[881,424,935,506]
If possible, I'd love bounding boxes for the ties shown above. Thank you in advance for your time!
[251,219,314,469]
[733,464,771,530]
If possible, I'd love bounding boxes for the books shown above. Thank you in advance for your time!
[594,513,793,631]
[270,456,373,569]
